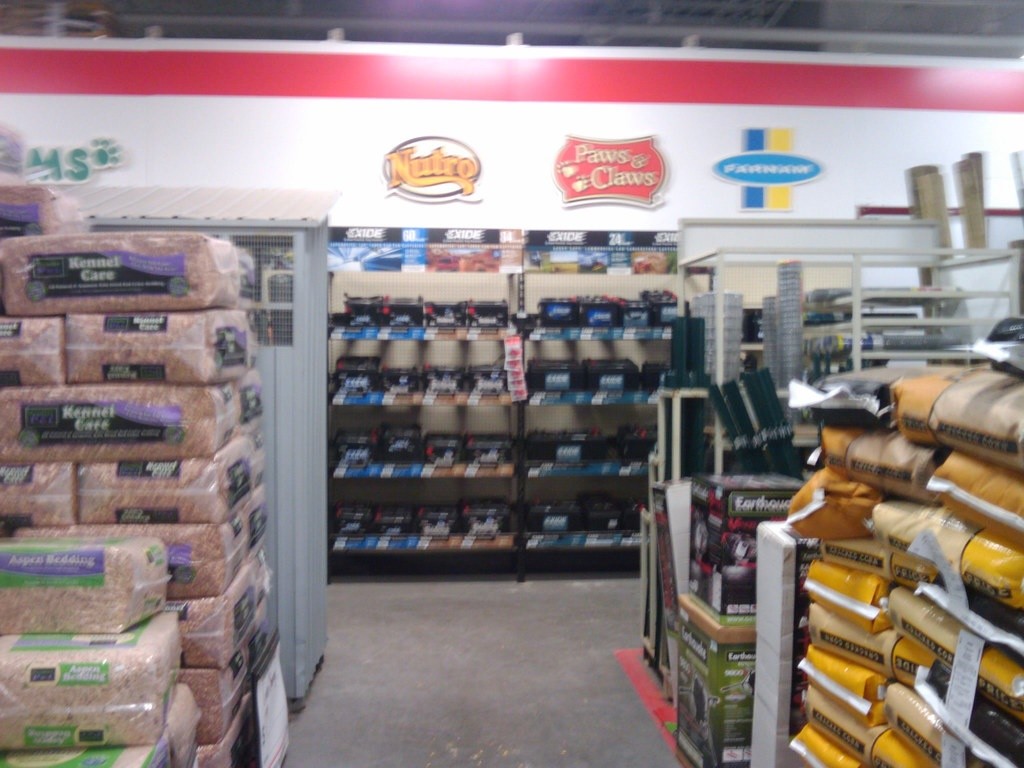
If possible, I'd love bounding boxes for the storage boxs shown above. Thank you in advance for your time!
[653,449,811,768]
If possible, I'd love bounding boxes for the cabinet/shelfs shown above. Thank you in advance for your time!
[328,220,1022,579]
